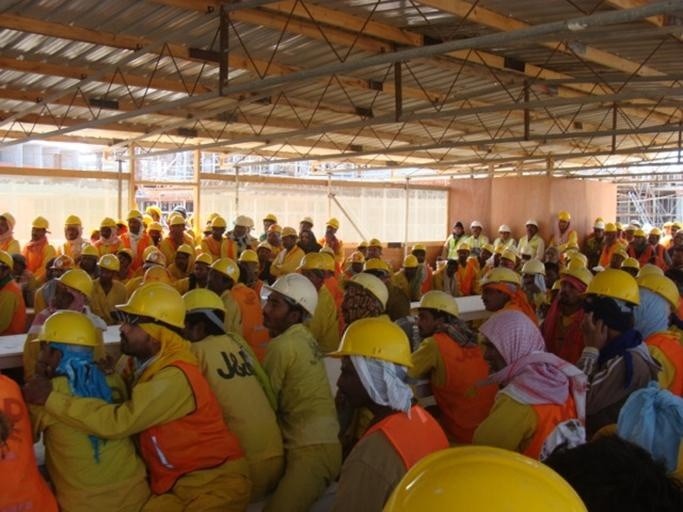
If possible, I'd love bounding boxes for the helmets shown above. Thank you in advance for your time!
[3,206,427,271]
[452,211,681,277]
[479,266,523,289]
[583,269,640,307]
[114,282,186,329]
[182,288,228,314]
[381,445,590,511]
[381,443,590,511]
[140,267,171,285]
[324,316,413,368]
[53,269,93,302]
[635,274,680,314]
[338,272,389,313]
[30,309,101,347]
[411,290,459,322]
[264,273,319,319]
[208,257,239,287]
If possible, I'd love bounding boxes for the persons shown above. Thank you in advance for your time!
[1,206,682,512]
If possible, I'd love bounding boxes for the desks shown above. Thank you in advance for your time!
[0,295,490,370]
